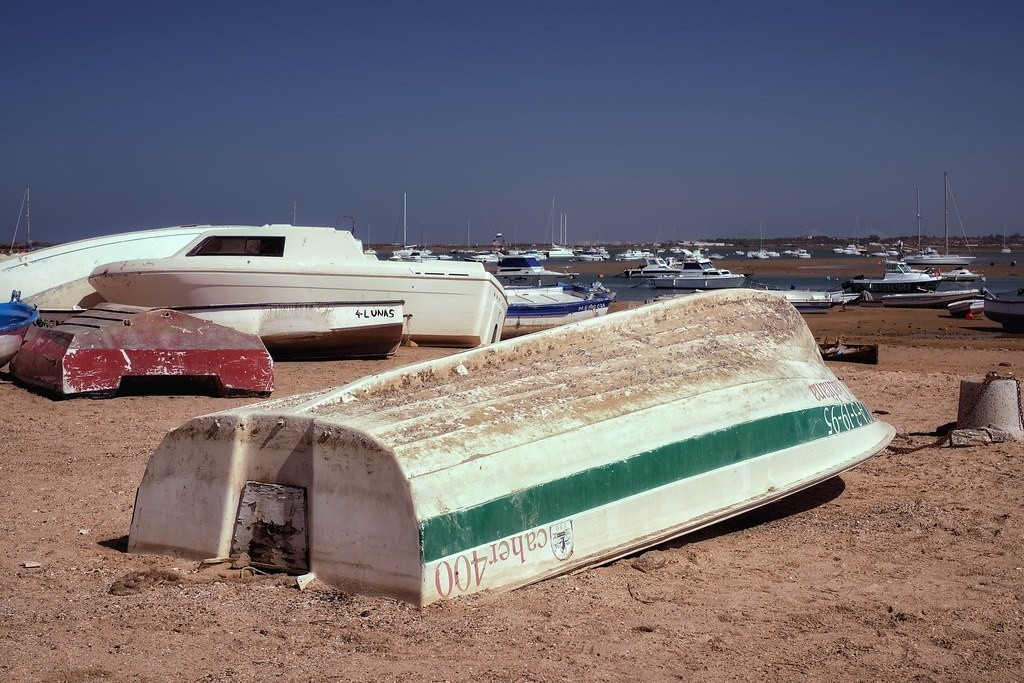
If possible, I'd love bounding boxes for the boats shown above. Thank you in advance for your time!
[0,172,1024,355]
[0,297,40,369]
[10,301,274,399]
[125,287,855,609]
[819,341,880,366]
